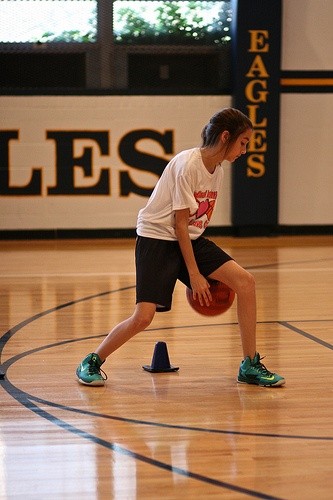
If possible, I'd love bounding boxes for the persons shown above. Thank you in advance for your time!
[75,107,285,388]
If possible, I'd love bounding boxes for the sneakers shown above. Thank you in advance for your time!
[75,353,107,386]
[236,353,285,387]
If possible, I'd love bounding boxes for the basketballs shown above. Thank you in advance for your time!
[186,277,235,317]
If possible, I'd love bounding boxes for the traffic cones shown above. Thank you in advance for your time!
[142,341,179,373]
